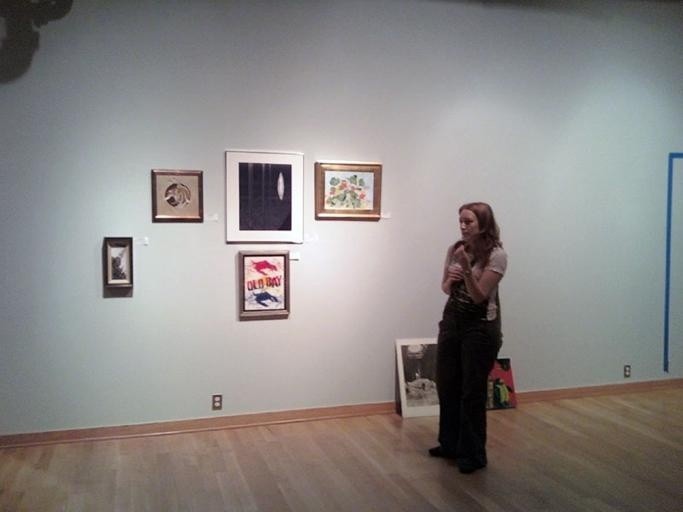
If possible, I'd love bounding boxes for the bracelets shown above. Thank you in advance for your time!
[462,269,473,279]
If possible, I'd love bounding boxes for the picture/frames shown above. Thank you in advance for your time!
[224,149,305,244]
[314,161,383,222]
[236,249,291,320]
[395,337,443,420]
[102,235,134,290]
[149,169,204,223]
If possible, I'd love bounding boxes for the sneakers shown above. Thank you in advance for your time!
[429,446,487,473]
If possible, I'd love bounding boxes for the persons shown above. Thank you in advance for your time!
[428,202,509,473]
